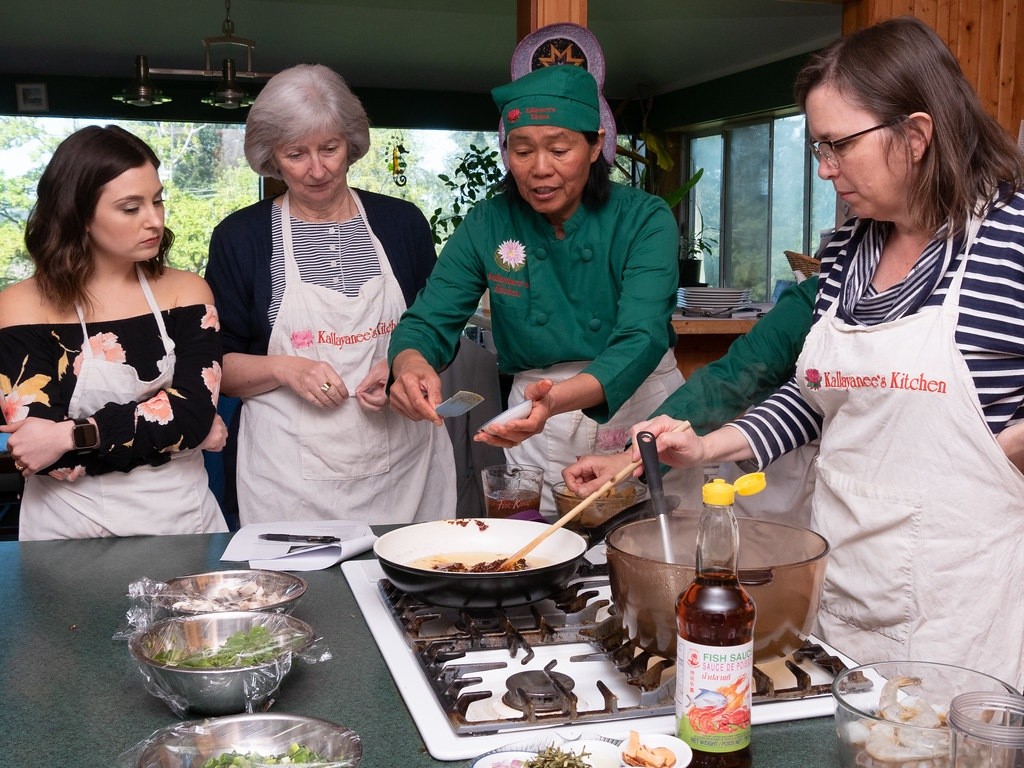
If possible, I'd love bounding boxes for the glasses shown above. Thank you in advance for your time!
[806,116,907,170]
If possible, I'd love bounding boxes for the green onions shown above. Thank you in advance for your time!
[200,741,324,768]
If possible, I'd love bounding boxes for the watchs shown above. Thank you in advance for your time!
[64,418,97,455]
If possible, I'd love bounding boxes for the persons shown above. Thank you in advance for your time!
[385,64,703,517]
[204,64,458,525]
[631,14,1023,695]
[0,124,229,540]
[561,275,822,501]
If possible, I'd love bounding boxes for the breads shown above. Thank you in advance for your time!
[620,730,677,768]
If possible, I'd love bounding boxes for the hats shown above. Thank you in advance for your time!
[491,65,600,142]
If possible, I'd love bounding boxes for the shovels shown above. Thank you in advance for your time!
[435,390,484,417]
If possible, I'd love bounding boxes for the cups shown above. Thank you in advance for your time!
[481,463,545,519]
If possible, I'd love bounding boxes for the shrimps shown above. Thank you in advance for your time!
[838,675,1006,768]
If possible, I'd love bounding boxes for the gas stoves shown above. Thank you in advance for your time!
[339,548,910,761]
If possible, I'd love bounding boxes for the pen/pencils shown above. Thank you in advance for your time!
[257,534,341,543]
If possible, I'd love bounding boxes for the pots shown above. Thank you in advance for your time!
[372,494,685,607]
[603,516,831,664]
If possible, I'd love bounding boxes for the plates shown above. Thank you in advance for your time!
[473,732,693,768]
[676,287,752,308]
[477,399,535,435]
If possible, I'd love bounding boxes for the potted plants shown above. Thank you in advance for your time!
[677,199,722,288]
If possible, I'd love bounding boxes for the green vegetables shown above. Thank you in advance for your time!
[523,740,593,768]
[150,625,279,669]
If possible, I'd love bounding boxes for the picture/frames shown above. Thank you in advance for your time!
[14,80,49,113]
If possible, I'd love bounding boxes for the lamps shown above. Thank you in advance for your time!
[111,0,262,108]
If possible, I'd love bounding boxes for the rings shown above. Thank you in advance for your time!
[15,460,27,471]
[311,397,317,405]
[320,382,331,393]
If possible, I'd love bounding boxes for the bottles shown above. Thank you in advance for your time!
[944,689,1024,768]
[675,471,768,768]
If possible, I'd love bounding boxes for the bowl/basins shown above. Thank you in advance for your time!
[831,660,1022,768]
[139,712,364,768]
[550,480,647,527]
[152,570,307,619]
[126,613,315,712]
